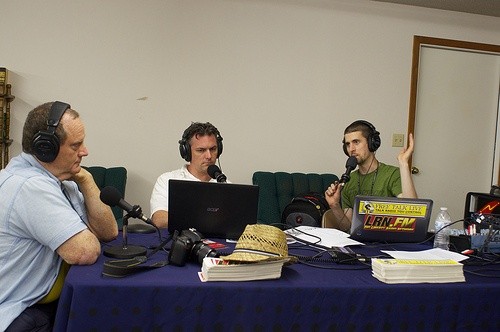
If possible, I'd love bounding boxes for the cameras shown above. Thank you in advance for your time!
[168,228,221,267]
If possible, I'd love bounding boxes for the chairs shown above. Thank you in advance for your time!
[81,165,127,232]
[252,171,340,230]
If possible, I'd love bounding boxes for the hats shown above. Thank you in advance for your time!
[219,224,299,266]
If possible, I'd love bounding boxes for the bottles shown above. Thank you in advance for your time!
[433,207,450,251]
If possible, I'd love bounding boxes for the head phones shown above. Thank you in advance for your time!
[179,122,223,162]
[343,120,381,156]
[32,101,71,161]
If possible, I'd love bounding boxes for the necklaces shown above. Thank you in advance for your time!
[357,160,378,196]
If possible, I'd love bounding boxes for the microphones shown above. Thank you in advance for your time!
[340,156,357,182]
[208,165,227,183]
[100,186,156,227]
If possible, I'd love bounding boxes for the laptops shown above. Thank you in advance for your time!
[168,179,259,239]
[348,196,433,243]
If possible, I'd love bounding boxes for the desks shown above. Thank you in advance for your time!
[51,227,500,332]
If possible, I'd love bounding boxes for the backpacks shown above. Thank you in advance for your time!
[280,192,330,232]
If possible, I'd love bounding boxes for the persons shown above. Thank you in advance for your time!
[0,101,119,332]
[324,121,417,232]
[149,122,232,230]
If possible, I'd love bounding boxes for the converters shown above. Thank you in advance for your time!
[331,246,357,264]
